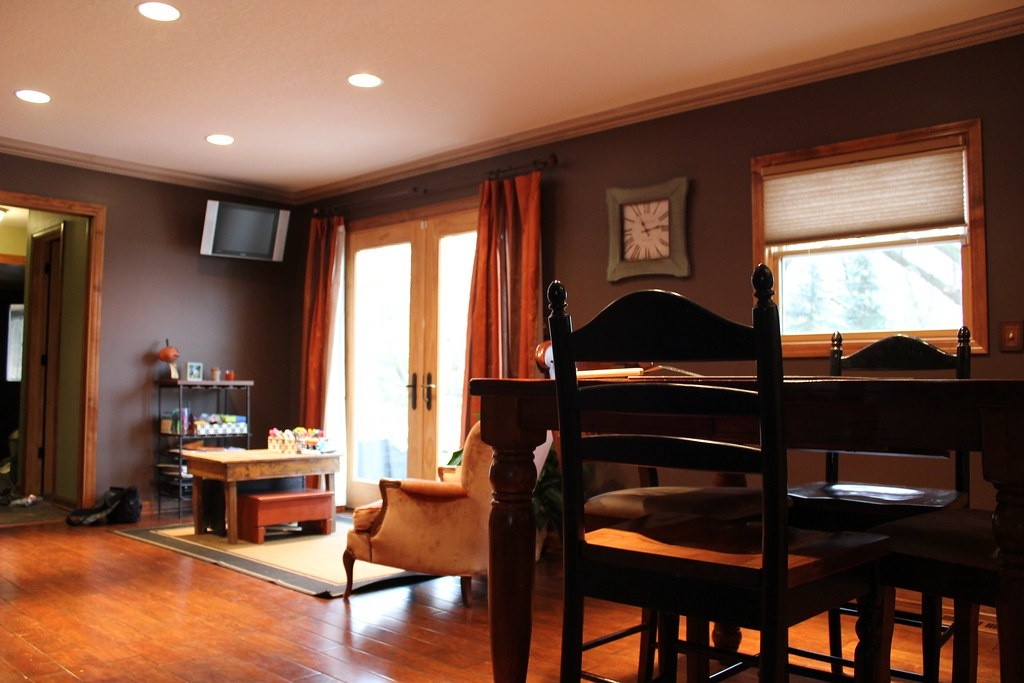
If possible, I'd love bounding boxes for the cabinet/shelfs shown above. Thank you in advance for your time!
[155,380,256,520]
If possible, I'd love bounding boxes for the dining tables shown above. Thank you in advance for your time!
[467,366,1024,683]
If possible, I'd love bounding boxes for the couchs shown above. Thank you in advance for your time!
[344,420,554,607]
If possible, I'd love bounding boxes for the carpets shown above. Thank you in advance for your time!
[115,513,446,599]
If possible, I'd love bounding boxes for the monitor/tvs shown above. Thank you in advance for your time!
[199,199,290,263]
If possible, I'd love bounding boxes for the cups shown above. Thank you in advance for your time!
[225,369,236,380]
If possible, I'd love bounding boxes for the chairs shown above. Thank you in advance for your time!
[785,326,973,683]
[545,262,897,683]
[533,340,764,683]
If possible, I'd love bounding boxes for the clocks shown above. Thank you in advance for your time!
[606,176,692,282]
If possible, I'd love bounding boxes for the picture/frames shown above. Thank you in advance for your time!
[186,362,204,382]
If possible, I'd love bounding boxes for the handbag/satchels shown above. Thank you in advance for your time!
[66,487,142,527]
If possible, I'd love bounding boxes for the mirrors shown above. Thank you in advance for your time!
[6,304,26,382]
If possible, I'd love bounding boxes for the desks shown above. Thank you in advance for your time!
[182,448,337,545]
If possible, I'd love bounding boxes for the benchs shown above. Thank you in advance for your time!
[238,490,335,545]
